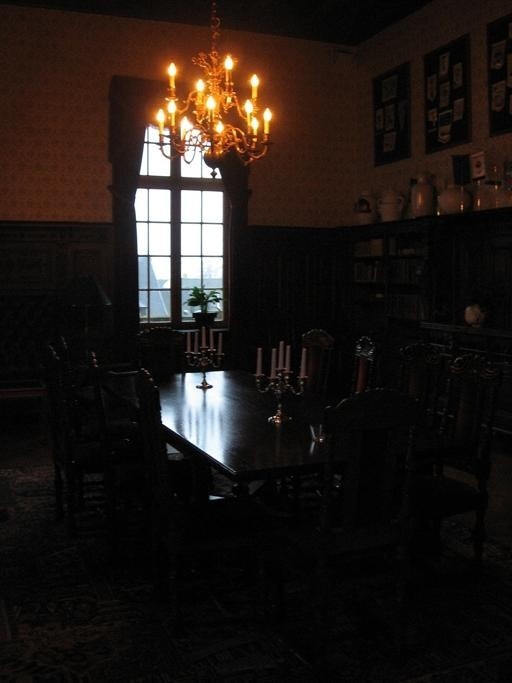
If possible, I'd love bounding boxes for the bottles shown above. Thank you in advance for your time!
[473,165,507,211]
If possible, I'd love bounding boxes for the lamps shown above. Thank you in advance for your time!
[151,2,276,183]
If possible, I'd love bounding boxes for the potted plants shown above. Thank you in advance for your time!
[186,286,224,323]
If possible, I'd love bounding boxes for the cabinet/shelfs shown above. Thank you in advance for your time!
[346,216,450,331]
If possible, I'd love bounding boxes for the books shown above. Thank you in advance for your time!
[256,227,511,401]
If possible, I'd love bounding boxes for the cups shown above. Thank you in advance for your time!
[309,422,331,440]
[308,441,328,456]
